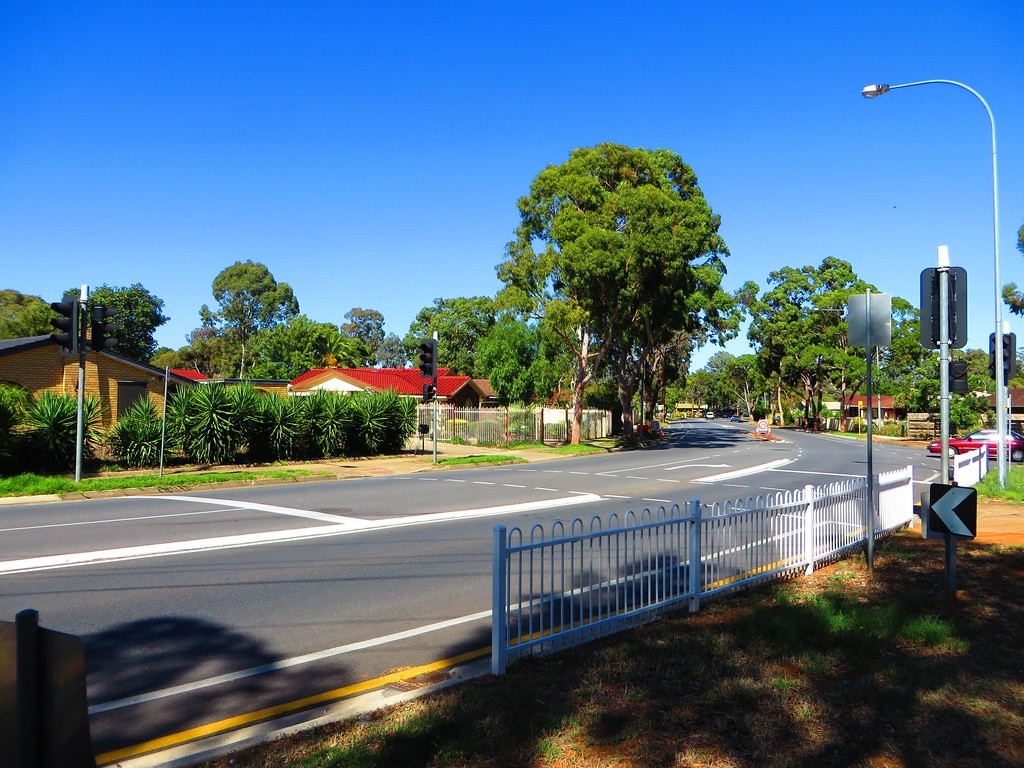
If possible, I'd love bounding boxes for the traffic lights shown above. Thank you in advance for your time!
[419,339,437,378]
[423,383,434,402]
[49,295,77,352]
[90,304,119,352]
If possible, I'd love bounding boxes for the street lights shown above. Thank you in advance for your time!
[861,79,1008,490]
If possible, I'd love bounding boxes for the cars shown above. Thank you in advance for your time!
[722,413,728,418]
[705,412,715,420]
[731,415,740,423]
[927,426,1024,462]
